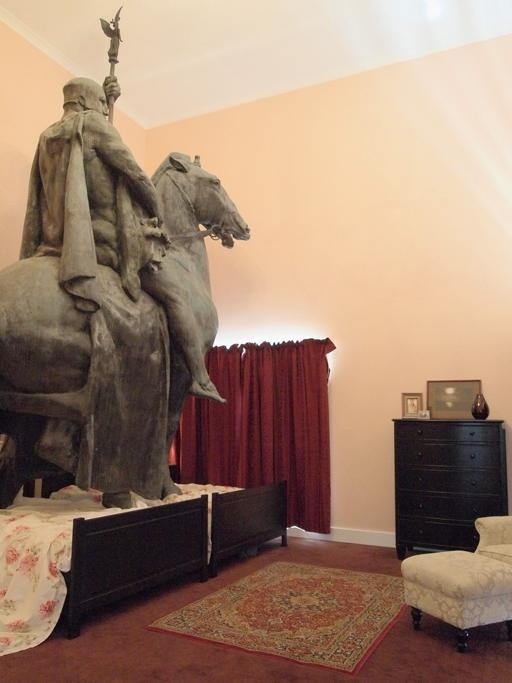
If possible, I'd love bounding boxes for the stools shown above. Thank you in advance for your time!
[400,549,511,653]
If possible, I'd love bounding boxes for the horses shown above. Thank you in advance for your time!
[1,149,252,510]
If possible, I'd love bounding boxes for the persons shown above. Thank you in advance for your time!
[407,398,417,413]
[20,76,231,405]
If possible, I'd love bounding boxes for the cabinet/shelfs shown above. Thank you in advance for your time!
[401,392,423,418]
[394,421,506,560]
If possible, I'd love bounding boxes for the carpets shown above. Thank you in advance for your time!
[143,559,406,678]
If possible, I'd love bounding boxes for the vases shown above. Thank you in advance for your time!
[471,393,489,420]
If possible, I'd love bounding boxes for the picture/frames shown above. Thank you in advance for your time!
[427,380,481,420]
